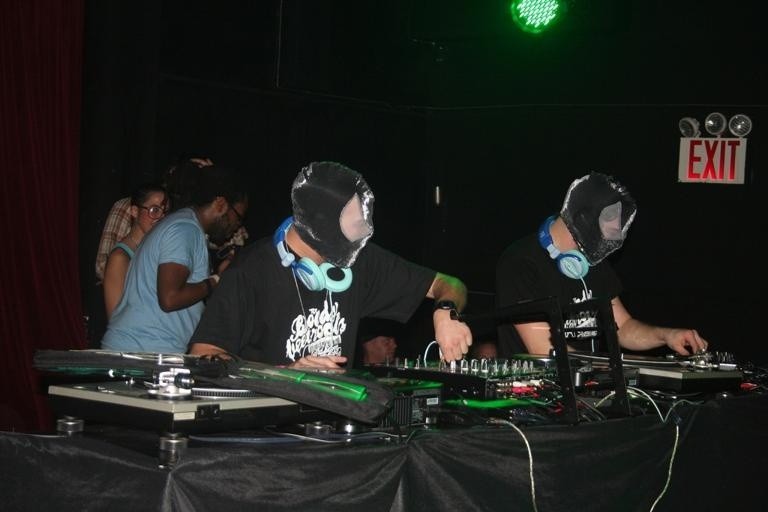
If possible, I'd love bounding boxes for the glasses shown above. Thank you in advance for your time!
[141,205,171,219]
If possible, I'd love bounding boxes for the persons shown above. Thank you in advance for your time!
[189,158,476,365]
[93,155,248,281]
[493,170,709,360]
[102,165,251,350]
[102,184,170,321]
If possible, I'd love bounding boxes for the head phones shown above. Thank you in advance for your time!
[538,215,589,279]
[275,217,354,291]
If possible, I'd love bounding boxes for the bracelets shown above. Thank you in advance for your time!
[202,278,212,295]
[211,274,220,285]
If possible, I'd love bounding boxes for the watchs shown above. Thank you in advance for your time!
[431,300,460,311]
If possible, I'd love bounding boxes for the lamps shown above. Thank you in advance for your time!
[677,110,754,139]
